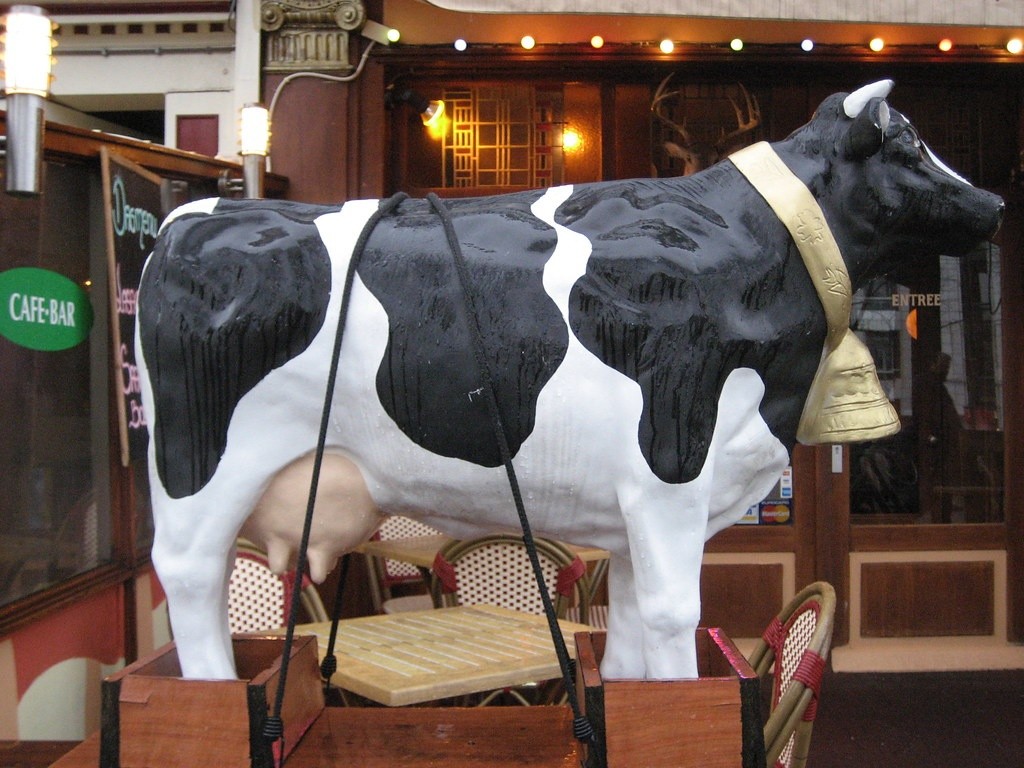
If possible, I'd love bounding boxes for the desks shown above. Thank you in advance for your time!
[353,537,611,614]
[267,604,604,708]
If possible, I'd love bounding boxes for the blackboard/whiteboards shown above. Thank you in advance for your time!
[101,146,171,467]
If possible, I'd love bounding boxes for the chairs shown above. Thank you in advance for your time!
[227,536,356,708]
[748,581,835,767]
[431,534,588,706]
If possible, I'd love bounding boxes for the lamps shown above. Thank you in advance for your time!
[385,83,445,125]
[0,4,58,197]
[217,103,272,200]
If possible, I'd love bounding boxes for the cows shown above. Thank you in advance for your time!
[133,79,1005,680]
